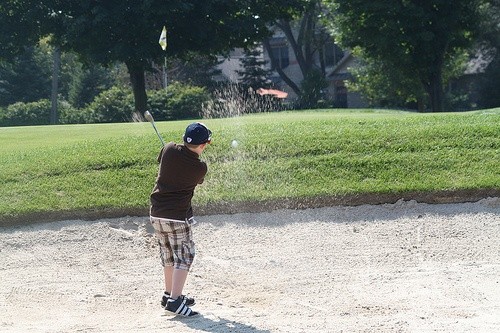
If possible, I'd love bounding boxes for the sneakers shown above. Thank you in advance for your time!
[160,291,195,308]
[164,296,199,318]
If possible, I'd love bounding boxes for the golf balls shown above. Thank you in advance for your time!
[232,140,239,150]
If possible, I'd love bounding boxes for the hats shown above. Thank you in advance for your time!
[184,122,212,144]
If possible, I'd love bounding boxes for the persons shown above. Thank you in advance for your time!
[149,123,213,317]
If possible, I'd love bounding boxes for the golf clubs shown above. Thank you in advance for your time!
[144,111,164,146]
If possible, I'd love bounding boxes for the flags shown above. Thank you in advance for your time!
[159,26,167,50]
